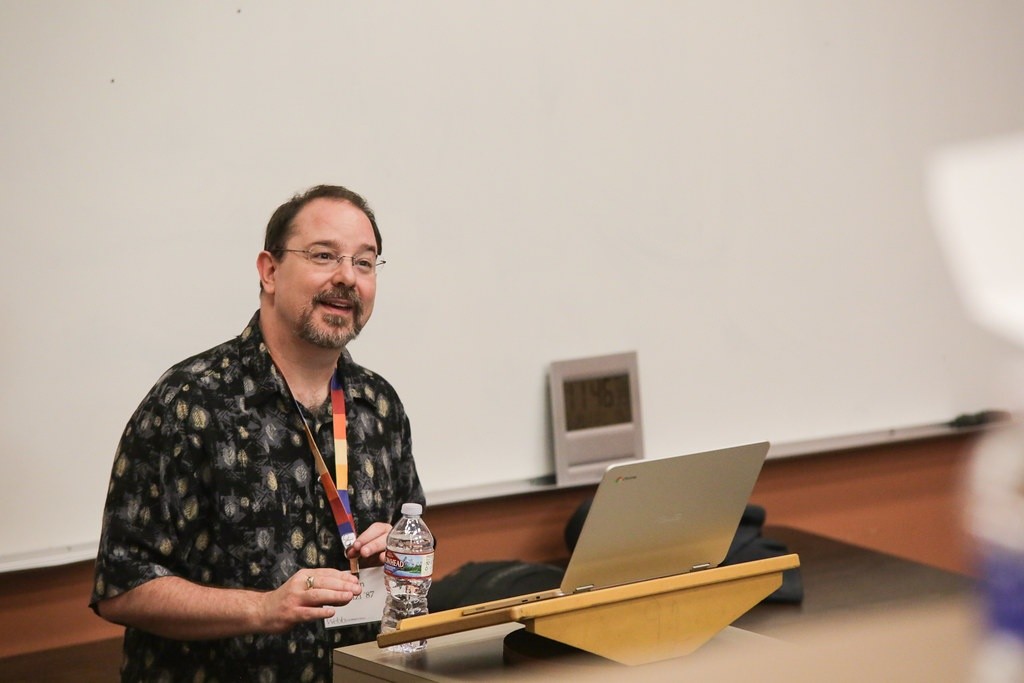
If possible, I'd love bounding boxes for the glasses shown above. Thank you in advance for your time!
[267,246,387,278]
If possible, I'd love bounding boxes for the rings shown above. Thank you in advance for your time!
[306,576,314,590]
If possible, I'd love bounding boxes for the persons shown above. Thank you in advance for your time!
[89,185,437,683]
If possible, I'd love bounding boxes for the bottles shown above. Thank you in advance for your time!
[380,502,434,654]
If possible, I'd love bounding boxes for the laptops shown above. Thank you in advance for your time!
[461,441,771,617]
